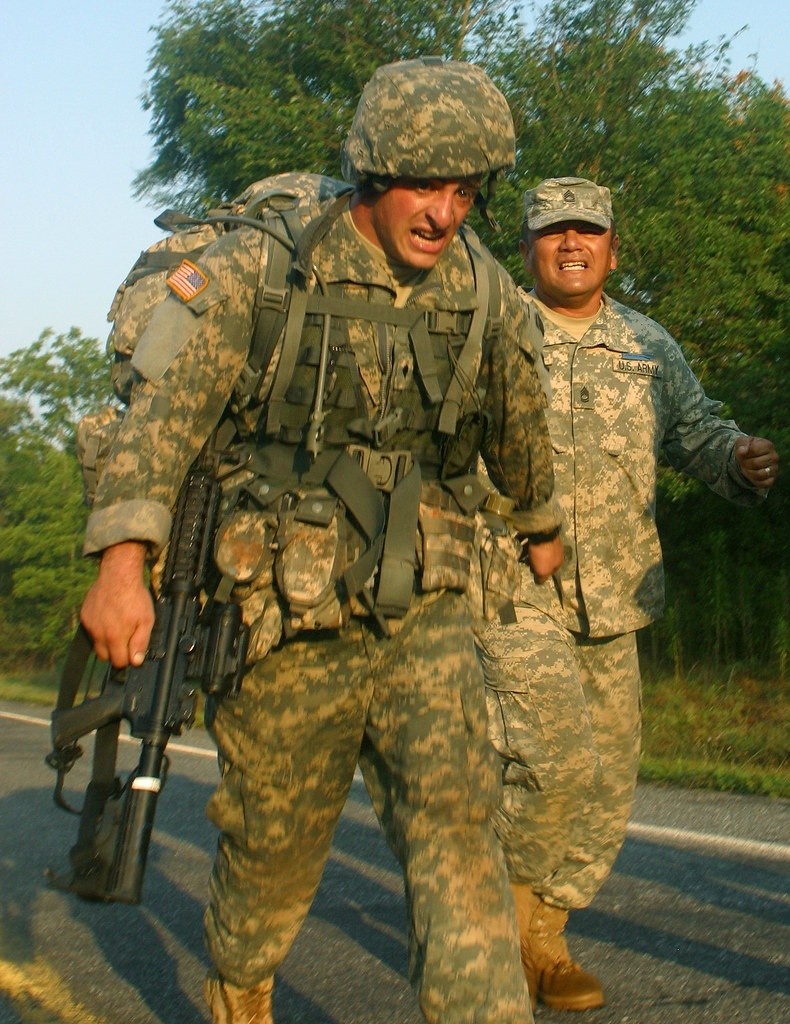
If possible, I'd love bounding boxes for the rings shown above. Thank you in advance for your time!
[765,467,770,478]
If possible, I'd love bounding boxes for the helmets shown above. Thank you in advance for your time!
[344,55,517,177]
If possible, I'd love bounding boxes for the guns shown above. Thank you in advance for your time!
[50,421,252,898]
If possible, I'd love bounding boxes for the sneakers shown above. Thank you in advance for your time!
[203,967,276,1024]
[510,884,606,1013]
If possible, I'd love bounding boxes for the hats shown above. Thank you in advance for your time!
[523,177,615,229]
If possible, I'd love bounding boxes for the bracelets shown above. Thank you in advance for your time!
[514,522,562,544]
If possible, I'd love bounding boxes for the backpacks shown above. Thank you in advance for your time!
[108,164,501,518]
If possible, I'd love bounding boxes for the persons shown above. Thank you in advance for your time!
[78,54,568,1023]
[471,178,779,1009]
